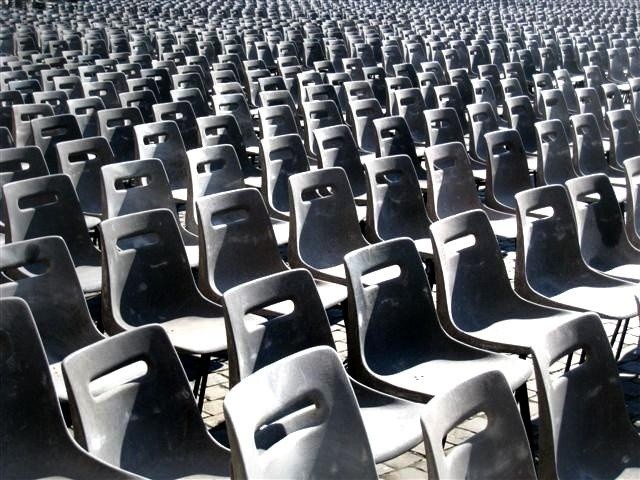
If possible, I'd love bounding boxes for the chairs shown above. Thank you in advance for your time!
[0,0,640,480]
